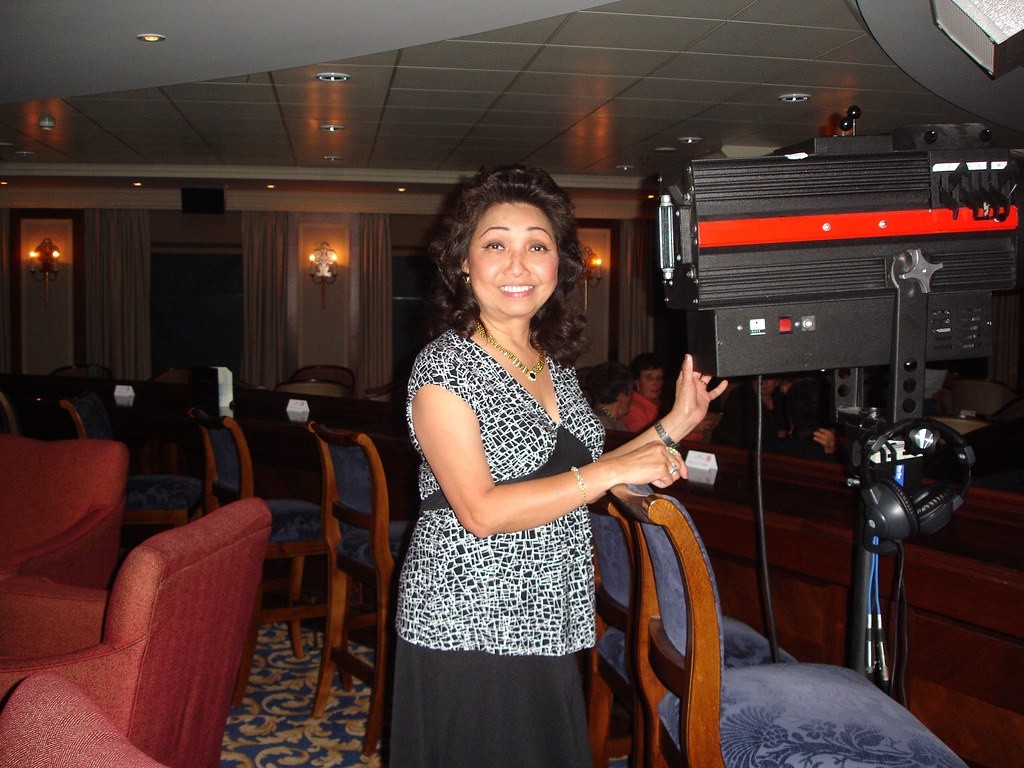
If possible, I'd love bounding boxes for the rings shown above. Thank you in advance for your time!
[700,378,708,385]
[670,462,678,477]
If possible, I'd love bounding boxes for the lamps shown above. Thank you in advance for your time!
[309,240,339,311]
[930,0,1024,82]
[580,246,602,315]
[28,238,62,311]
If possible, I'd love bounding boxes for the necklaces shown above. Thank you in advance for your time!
[473,321,546,382]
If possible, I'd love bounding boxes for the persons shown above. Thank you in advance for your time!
[386,163,730,768]
[587,352,836,462]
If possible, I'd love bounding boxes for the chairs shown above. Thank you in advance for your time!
[0,671,170,768]
[585,498,798,768]
[0,433,130,592]
[310,423,419,759]
[608,483,968,768]
[289,365,355,398]
[149,365,258,390]
[50,364,113,379]
[60,393,205,527]
[275,380,348,397]
[190,406,355,708]
[0,389,23,435]
[0,497,272,768]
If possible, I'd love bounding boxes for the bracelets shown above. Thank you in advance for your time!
[653,420,681,451]
[571,464,589,512]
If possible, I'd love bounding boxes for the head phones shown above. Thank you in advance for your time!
[858,415,975,545]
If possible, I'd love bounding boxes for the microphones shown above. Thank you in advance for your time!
[863,519,898,555]
